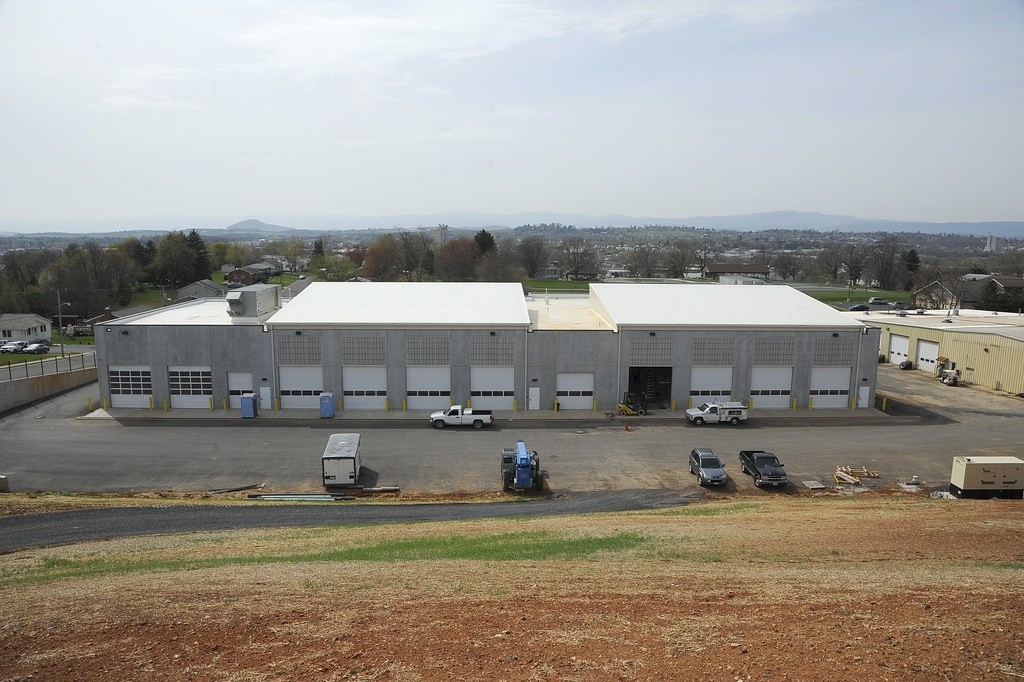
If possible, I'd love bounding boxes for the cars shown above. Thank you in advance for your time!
[894,302,911,310]
[868,297,887,304]
[22,343,50,354]
[849,304,869,311]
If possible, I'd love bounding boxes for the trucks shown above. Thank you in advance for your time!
[62,324,92,336]
[320,432,362,488]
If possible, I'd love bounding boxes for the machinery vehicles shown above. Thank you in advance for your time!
[499,439,543,492]
[617,390,647,417]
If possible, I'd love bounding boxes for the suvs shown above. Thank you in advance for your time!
[688,445,729,488]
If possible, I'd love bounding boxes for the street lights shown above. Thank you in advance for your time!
[59,302,70,357]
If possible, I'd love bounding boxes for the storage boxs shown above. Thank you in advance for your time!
[463,407,472,415]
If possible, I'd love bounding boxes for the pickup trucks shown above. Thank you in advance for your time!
[739,451,789,490]
[683,400,748,426]
[428,403,494,430]
[0,340,30,354]
